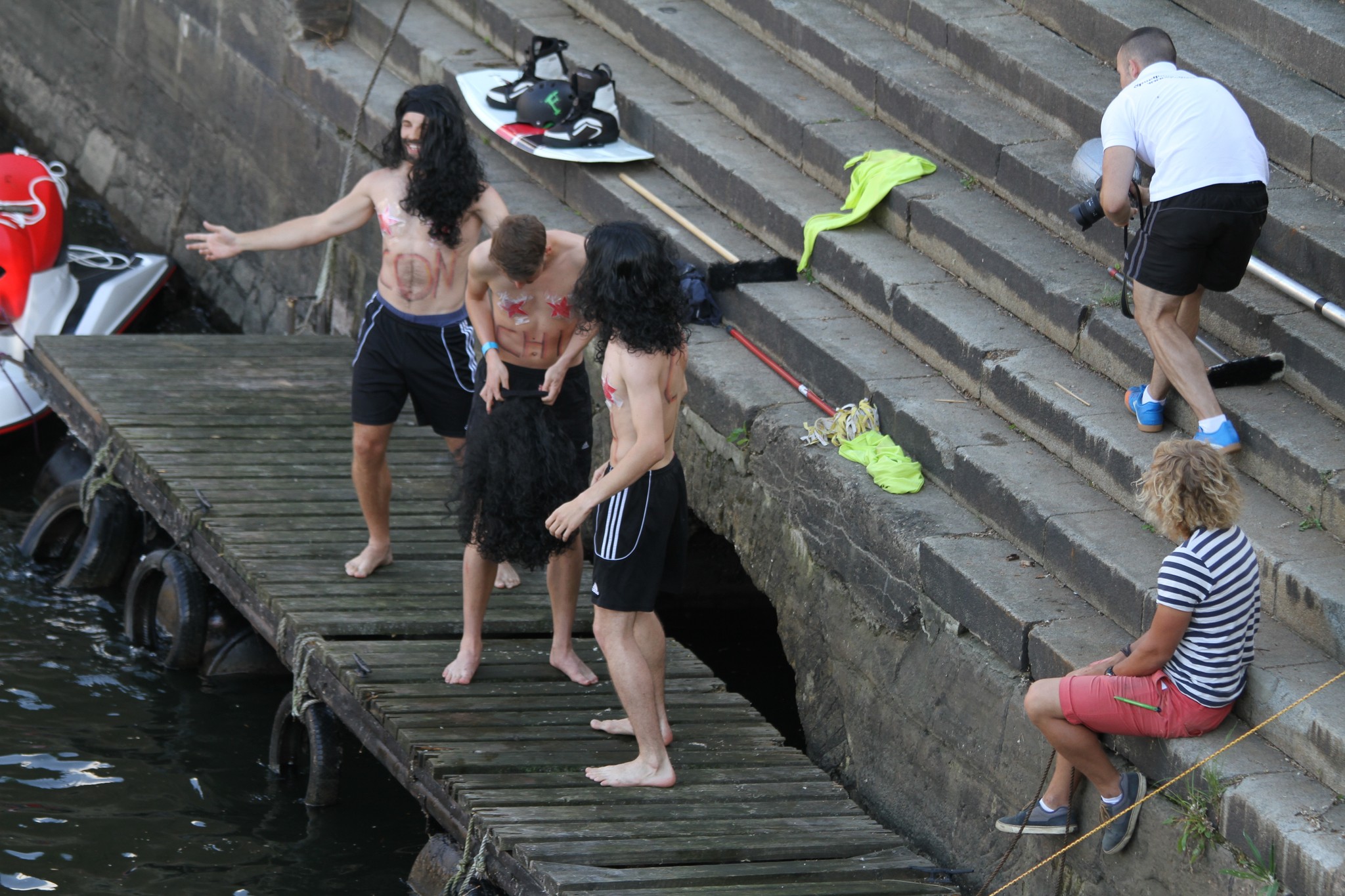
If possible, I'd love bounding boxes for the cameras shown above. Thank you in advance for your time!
[1068,173,1136,233]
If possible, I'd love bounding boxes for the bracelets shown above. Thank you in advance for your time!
[481,342,499,357]
[1121,644,1132,657]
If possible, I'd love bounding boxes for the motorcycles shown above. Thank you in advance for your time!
[0,146,174,469]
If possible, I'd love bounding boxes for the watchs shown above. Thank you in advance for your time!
[1104,666,1115,677]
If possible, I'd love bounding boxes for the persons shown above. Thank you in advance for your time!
[992,440,1261,853]
[544,220,689,789]
[184,85,523,589]
[440,214,604,686]
[1091,26,1270,457]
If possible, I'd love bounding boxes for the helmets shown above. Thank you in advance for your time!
[515,78,574,128]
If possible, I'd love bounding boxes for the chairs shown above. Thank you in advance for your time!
[0,153,63,321]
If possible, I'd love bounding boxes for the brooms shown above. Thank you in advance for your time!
[1104,264,1285,387]
[617,169,801,287]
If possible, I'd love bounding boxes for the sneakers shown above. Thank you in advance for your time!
[543,68,620,147]
[487,36,568,109]
[1125,383,1164,433]
[1101,772,1146,853]
[1192,419,1241,456]
[995,798,1077,835]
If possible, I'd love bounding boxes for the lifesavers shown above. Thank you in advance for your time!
[17,478,142,596]
[268,693,351,805]
[123,548,200,679]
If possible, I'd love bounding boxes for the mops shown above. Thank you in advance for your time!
[723,315,884,449]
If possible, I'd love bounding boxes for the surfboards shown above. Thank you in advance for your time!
[455,34,656,165]
